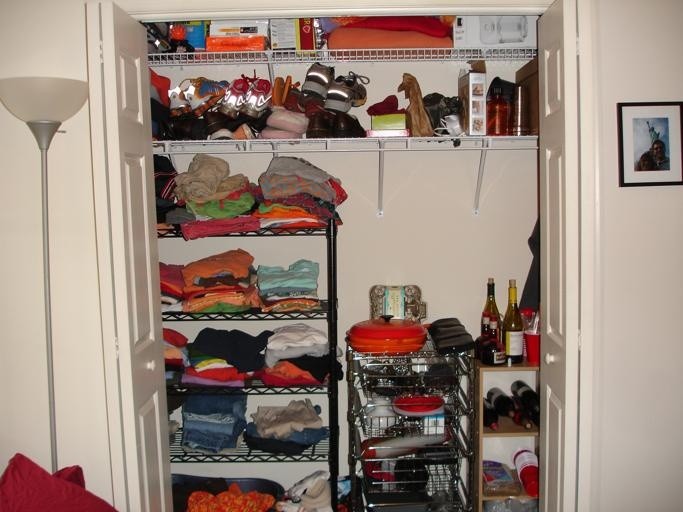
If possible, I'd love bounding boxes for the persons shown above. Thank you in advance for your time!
[636,140,669,170]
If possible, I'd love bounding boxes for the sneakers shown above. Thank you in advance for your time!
[167,62,369,140]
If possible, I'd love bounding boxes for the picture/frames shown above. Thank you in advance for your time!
[614,101,682,189]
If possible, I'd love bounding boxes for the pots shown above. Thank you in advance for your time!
[344,313,432,354]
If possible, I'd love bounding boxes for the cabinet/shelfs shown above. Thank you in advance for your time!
[469,353,538,512]
[340,349,467,512]
[156,218,342,511]
[139,15,538,214]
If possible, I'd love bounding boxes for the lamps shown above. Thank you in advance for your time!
[1,72,94,476]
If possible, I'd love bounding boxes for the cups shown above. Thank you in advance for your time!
[521,329,541,366]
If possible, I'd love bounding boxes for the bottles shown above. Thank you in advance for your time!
[484,86,510,135]
[510,84,531,135]
[474,275,524,366]
[483,379,539,431]
[513,449,541,496]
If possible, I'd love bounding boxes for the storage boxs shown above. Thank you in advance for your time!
[513,54,541,135]
